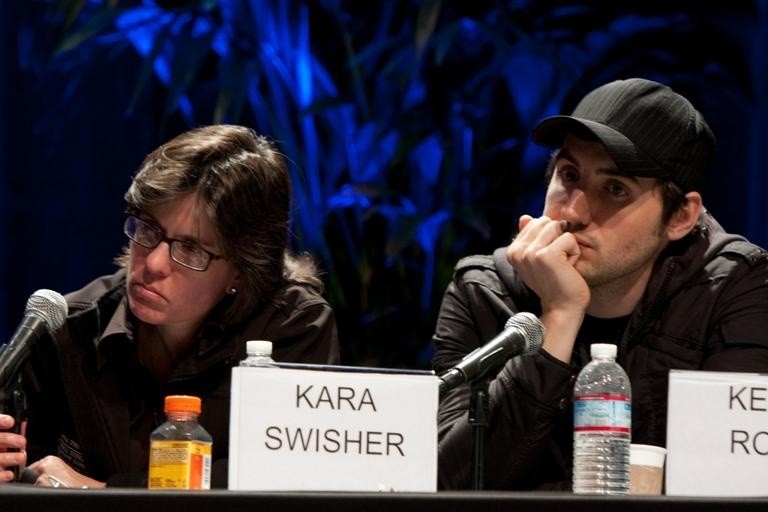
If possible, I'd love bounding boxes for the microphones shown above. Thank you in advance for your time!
[436,311,547,391]
[0,288,69,389]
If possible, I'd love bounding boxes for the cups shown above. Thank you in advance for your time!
[629,442,671,495]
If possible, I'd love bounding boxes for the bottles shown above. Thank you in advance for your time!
[145,393,215,490]
[568,344,635,496]
[237,339,280,370]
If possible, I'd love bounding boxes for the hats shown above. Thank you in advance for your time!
[531,77,721,192]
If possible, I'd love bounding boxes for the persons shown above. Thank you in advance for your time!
[433,77,767,493]
[0,124,342,491]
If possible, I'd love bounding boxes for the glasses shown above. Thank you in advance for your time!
[121,205,231,273]
